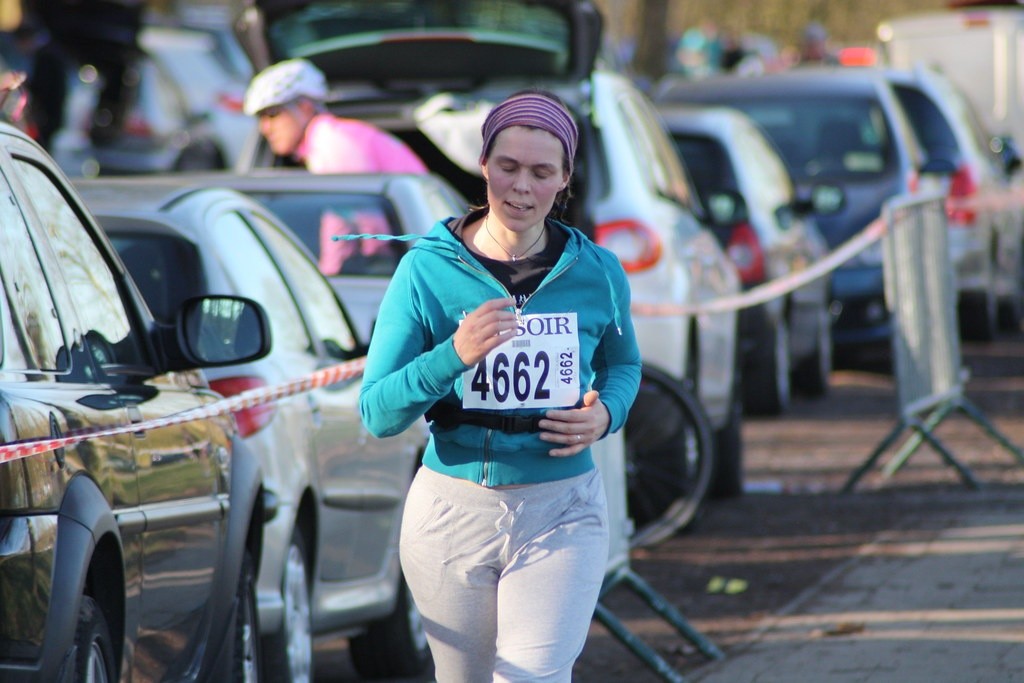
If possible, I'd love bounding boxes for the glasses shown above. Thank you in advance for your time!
[258,98,305,119]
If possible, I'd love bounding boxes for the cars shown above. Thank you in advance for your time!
[23,0,1024,534]
[69,175,443,683]
[0,126,279,683]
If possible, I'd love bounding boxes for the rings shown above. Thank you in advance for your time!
[577,435,581,442]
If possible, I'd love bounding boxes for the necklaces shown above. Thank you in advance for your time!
[485,214,545,261]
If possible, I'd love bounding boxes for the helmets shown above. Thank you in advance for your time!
[242,57,329,116]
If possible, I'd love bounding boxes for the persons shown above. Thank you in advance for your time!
[357,92,643,683]
[245,57,431,276]
[19,0,147,152]
[799,21,839,66]
[673,18,745,76]
